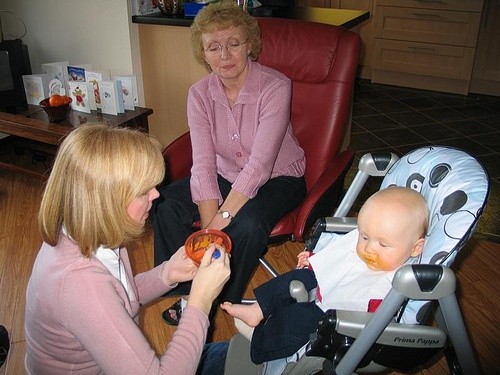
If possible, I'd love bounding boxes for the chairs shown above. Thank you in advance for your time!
[162,16,361,306]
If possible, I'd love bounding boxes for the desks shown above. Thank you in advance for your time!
[0,102,153,181]
[132,8,370,152]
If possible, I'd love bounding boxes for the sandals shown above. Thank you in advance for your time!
[161,298,188,327]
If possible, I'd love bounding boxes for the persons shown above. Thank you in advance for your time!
[219,186,430,365]
[23,125,231,375]
[152,0,308,330]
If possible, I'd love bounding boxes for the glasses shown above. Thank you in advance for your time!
[202,37,248,55]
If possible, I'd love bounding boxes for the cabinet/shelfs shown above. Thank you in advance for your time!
[330,0,500,96]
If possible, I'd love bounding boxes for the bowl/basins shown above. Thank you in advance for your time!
[39,95,71,122]
[185,228,232,268]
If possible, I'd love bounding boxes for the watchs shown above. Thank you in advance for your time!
[216,209,234,224]
